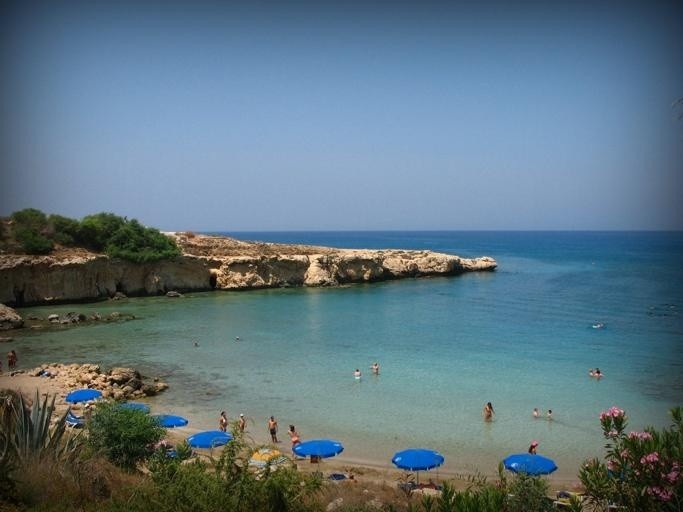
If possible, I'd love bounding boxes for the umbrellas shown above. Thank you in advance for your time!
[390,448,444,485]
[149,413,190,429]
[291,439,343,473]
[111,400,151,415]
[501,452,557,478]
[64,388,103,402]
[185,430,234,458]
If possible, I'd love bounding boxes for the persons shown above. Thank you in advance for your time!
[418,476,444,491]
[531,407,541,420]
[527,441,539,456]
[239,413,245,435]
[352,369,361,380]
[218,411,226,432]
[286,424,298,458]
[367,362,379,374]
[82,404,92,426]
[267,415,278,444]
[481,401,495,421]
[222,415,227,432]
[5,349,14,371]
[12,352,17,366]
[588,366,604,377]
[545,409,552,421]
